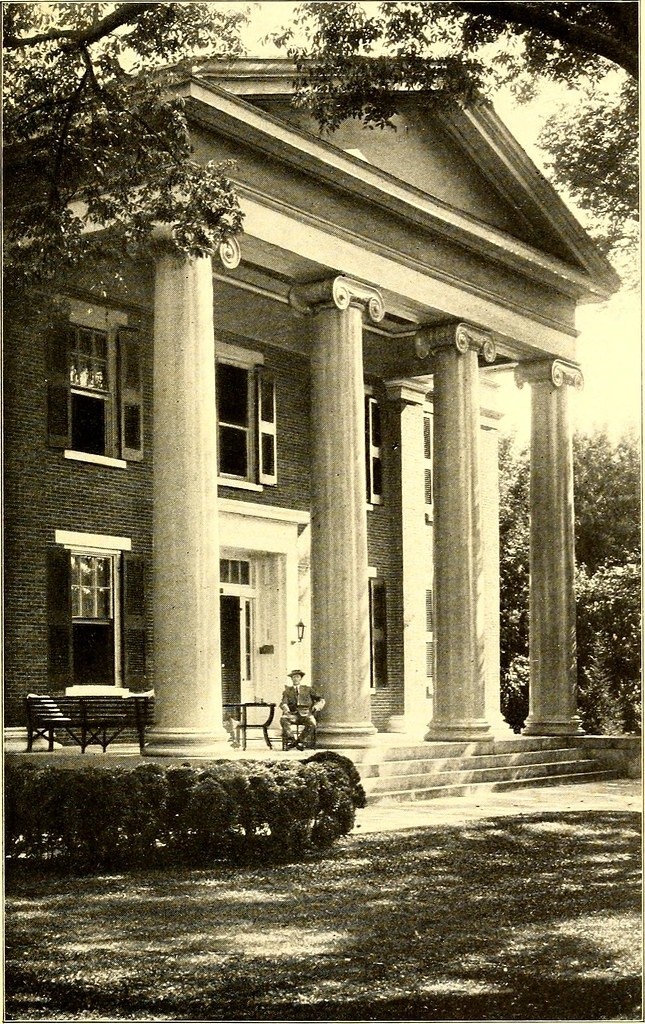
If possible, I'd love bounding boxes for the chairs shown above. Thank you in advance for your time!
[282,696,321,749]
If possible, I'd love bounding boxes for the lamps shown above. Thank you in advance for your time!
[297,619,305,643]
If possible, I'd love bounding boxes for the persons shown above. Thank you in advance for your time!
[280,669,326,751]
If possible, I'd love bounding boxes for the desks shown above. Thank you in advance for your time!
[225,701,276,749]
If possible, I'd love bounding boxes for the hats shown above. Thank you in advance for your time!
[287,670,305,678]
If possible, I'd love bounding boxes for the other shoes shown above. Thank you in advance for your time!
[283,740,305,752]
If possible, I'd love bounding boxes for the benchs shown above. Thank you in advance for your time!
[24,693,156,755]
